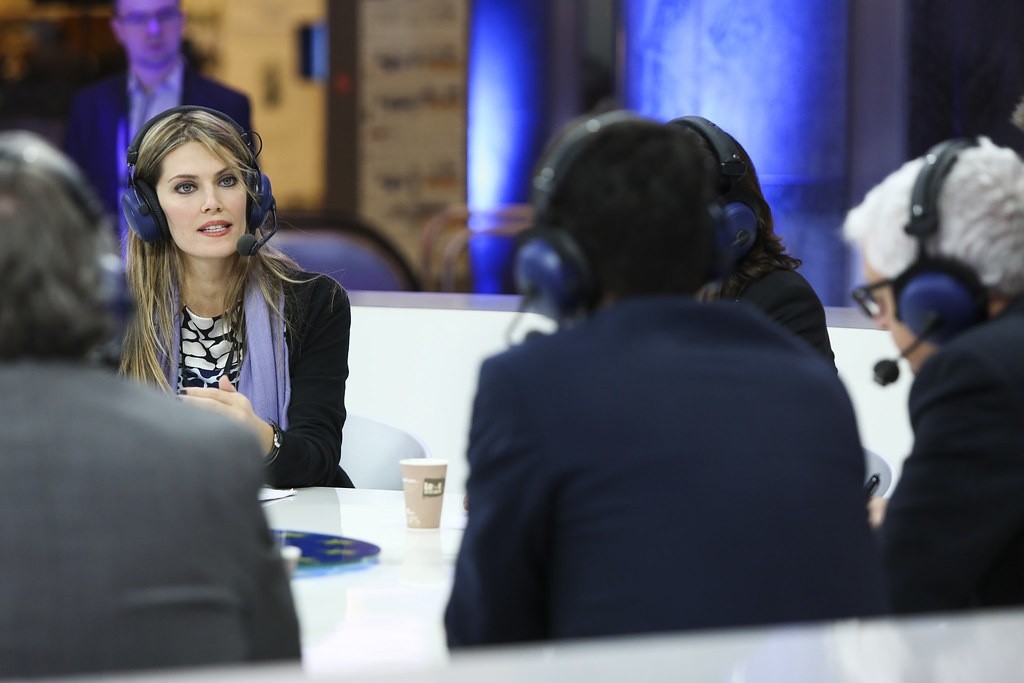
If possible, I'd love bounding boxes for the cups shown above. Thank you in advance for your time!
[400,458,448,529]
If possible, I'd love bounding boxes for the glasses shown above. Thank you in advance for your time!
[117,7,178,24]
[852,278,894,318]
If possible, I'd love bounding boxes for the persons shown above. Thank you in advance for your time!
[0,131,303,683]
[58,0,259,235]
[443,110,880,666]
[835,135,1024,617]
[103,105,357,490]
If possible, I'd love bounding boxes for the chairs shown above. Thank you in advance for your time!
[342,410,431,494]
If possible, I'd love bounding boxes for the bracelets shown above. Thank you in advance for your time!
[260,418,283,468]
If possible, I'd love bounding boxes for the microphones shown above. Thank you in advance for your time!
[509,289,544,347]
[237,198,277,257]
[874,309,945,387]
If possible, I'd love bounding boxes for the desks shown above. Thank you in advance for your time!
[258,484,468,656]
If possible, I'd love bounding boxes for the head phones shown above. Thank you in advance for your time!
[895,137,990,345]
[123,105,276,242]
[519,111,758,324]
[0,130,137,361]
